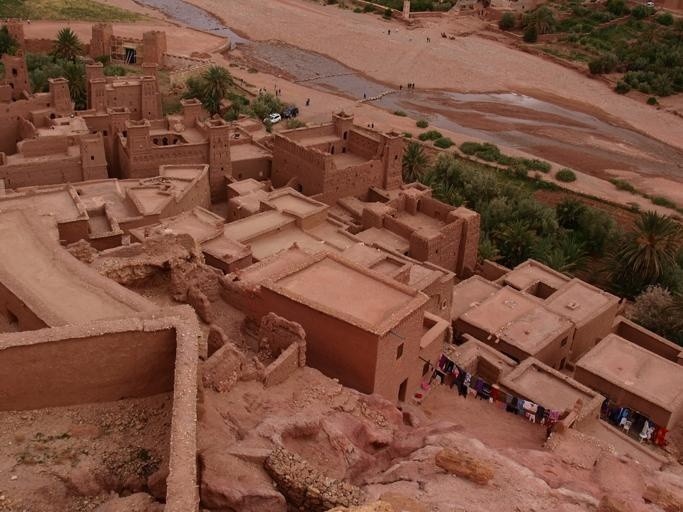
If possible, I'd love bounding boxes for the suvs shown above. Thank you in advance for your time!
[281,107,298,118]
[263,112,280,125]
